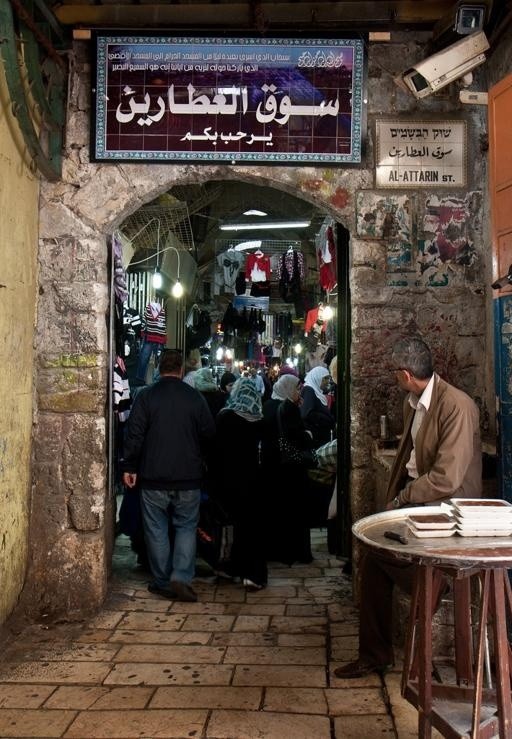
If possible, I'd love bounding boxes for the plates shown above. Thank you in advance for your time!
[404,498,512,538]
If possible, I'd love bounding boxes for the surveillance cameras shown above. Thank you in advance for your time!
[435,6,482,36]
[393,30,490,105]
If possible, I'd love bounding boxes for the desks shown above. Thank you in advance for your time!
[351,503,512,738]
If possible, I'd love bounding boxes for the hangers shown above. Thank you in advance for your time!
[123,302,129,309]
[255,247,262,255]
[318,302,324,310]
[287,246,293,252]
[227,245,234,252]
[152,296,156,303]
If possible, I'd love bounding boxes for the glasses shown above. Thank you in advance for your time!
[388,367,412,377]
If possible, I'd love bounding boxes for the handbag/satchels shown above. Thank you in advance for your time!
[277,400,319,466]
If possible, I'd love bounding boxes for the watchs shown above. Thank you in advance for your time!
[392,497,400,508]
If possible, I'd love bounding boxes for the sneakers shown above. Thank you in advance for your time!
[195,554,262,592]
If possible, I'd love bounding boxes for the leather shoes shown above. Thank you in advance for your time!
[169,581,197,603]
[334,656,394,679]
[148,580,171,599]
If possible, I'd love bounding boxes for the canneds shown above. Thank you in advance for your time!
[379,414,390,441]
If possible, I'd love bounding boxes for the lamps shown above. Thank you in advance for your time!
[120,218,162,290]
[126,247,182,298]
[219,221,311,231]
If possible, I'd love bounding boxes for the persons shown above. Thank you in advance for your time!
[334,336,484,679]
[120,348,215,605]
[120,366,339,590]
